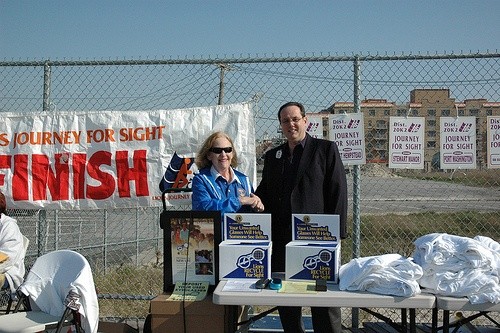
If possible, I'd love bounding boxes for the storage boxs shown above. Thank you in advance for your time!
[219,212,273,281]
[285,213,342,284]
[148,292,229,333]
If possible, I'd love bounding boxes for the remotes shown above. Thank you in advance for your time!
[269,277,282,290]
[256,279,269,289]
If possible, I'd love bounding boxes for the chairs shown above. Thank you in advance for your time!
[0,249,100,333]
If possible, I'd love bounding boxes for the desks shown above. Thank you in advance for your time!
[212,280,500,333]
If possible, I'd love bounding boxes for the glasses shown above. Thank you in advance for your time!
[209,147,232,154]
[280,117,303,126]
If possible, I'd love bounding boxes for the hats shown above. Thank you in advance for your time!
[0,191,6,209]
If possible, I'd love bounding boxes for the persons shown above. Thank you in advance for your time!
[172,222,213,275]
[0,189,25,293]
[192,132,258,333]
[250,102,348,333]
